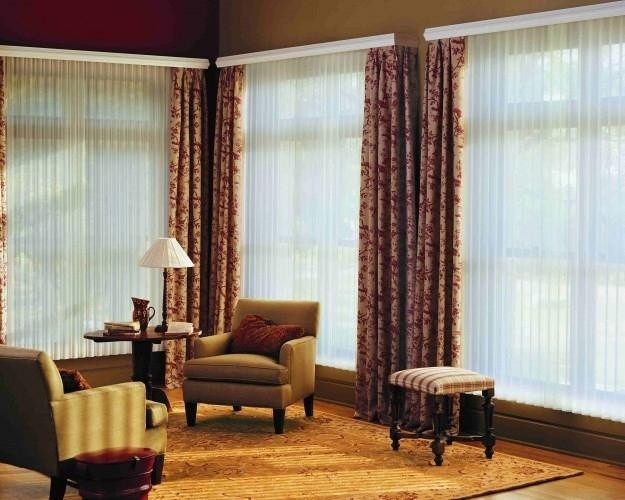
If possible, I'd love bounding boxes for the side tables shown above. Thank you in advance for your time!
[83,320,193,415]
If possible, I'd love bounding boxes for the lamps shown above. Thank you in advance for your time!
[139,236,194,331]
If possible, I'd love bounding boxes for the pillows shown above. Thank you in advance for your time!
[58,367,91,394]
[228,313,306,357]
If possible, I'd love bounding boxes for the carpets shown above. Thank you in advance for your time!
[0,391,584,500]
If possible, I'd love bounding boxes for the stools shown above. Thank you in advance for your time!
[387,365,498,466]
[69,446,157,500]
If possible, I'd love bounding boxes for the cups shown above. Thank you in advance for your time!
[131,297,155,332]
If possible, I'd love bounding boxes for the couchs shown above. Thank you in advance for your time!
[0,347,170,499]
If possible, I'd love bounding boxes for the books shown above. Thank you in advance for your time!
[102,319,142,337]
[164,320,194,337]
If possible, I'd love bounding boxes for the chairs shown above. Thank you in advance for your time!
[181,296,321,434]
